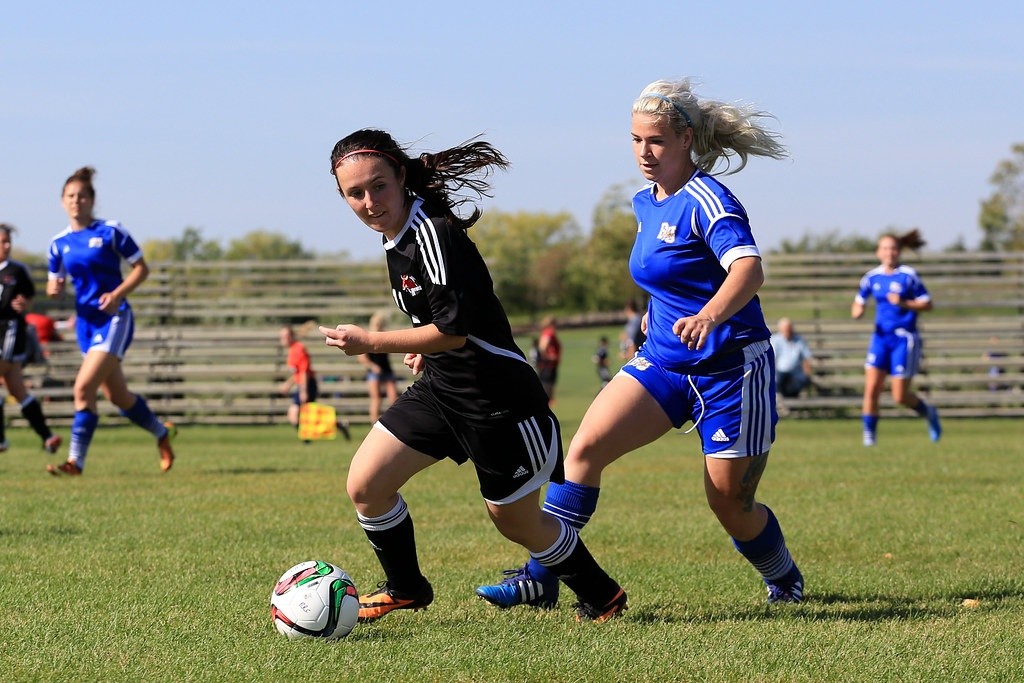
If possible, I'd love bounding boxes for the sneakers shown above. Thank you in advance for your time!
[926,408,945,441]
[569,582,631,629]
[476,561,558,613]
[47,459,84,477]
[158,421,179,475]
[0,441,10,451]
[44,434,64,454]
[354,579,435,623]
[862,428,876,449]
[764,565,805,606]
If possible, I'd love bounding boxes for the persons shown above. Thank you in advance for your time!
[47,165,177,477]
[318,127,629,623]
[475,74,804,610]
[537,311,562,404]
[0,223,62,453]
[771,315,815,419]
[357,313,401,427]
[279,326,352,443]
[593,335,612,391]
[850,227,942,447]
[985,334,1013,393]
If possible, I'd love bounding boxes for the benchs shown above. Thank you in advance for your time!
[758,251,1024,418]
[5,265,419,426]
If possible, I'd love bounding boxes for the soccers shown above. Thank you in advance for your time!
[271,562,360,642]
